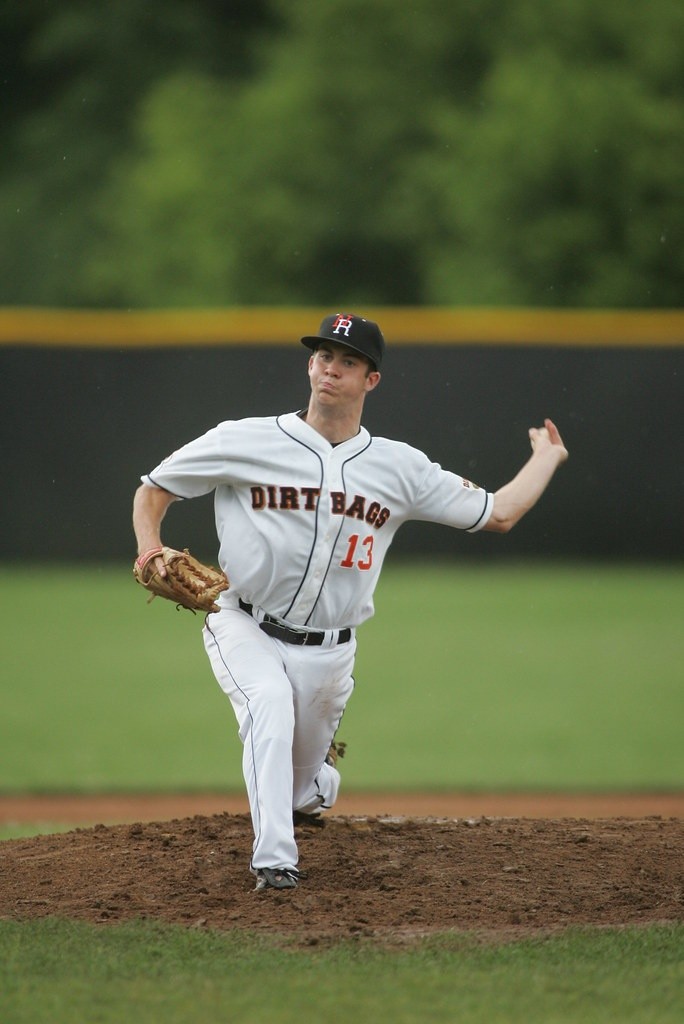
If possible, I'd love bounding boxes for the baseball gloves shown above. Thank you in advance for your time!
[132,547,230,615]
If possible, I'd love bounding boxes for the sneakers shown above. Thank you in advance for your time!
[292,810,325,835]
[256,868,308,890]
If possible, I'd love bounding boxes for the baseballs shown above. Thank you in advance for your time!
[537,427,550,440]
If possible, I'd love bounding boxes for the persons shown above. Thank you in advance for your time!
[132,312,568,889]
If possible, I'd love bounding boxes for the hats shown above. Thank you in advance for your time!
[301,314,385,373]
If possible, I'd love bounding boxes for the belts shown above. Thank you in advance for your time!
[239,598,351,646]
